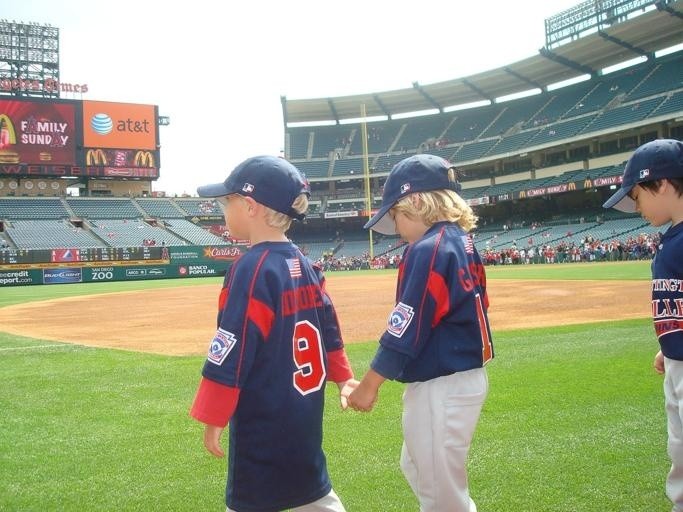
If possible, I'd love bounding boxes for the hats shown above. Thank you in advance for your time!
[194,154,312,226]
[601,138,683,215]
[360,151,462,239]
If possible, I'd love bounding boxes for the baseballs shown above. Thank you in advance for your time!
[92,114,114,134]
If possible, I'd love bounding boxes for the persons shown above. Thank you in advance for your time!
[472,200,660,265]
[433,137,448,154]
[190,153,362,511]
[622,66,640,75]
[602,139,683,512]
[346,152,496,512]
[299,215,401,270]
[534,117,556,135]
[579,84,640,112]
[142,237,165,246]
[332,124,410,175]
